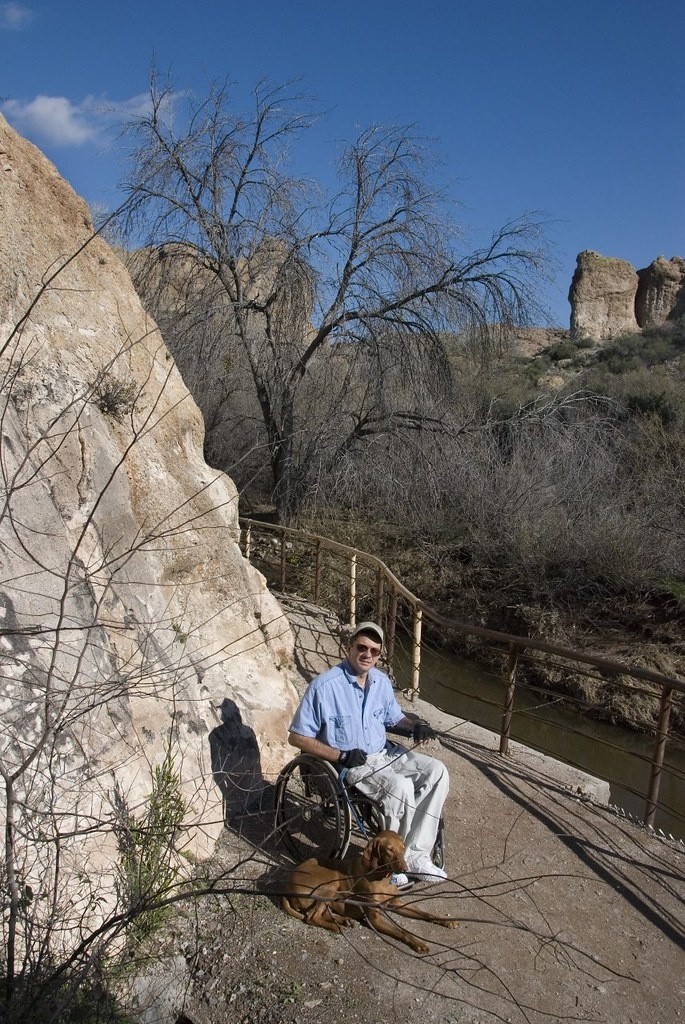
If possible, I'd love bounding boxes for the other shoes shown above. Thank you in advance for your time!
[405,862,447,882]
[391,873,408,886]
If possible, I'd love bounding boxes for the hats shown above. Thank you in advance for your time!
[350,621,383,645]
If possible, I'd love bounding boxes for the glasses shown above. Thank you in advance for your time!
[352,642,381,657]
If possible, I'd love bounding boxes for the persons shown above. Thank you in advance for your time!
[288,621,449,885]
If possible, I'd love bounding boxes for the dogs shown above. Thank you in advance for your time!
[280,830,463,956]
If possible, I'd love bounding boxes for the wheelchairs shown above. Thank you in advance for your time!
[274,726,446,890]
